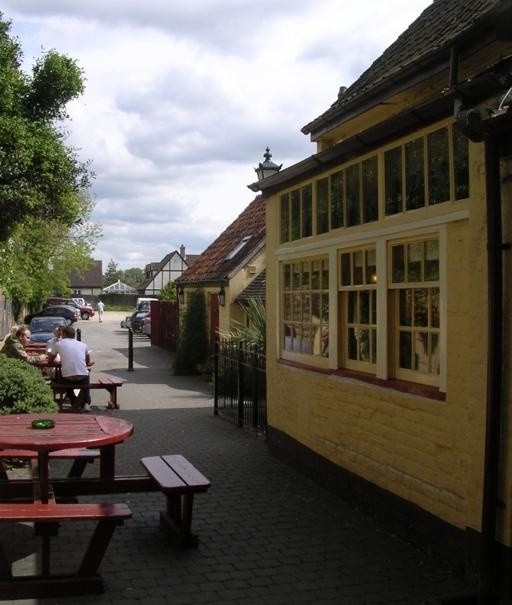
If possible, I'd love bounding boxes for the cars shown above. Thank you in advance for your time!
[28,316,69,343]
[131,296,159,336]
[24,304,76,326]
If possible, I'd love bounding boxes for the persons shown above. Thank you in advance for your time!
[45,327,63,364]
[321,327,329,357]
[0,327,47,366]
[284,324,308,354]
[48,326,97,413]
[96,300,106,322]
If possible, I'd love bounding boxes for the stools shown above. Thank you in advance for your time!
[140,456,213,549]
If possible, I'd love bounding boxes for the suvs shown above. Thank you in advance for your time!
[42,297,95,320]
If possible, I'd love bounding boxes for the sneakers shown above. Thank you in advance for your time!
[76,403,93,412]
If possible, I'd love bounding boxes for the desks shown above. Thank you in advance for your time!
[0,413,134,540]
[35,355,95,367]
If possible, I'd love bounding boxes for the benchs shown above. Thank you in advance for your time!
[25,343,49,355]
[1,450,101,480]
[0,503,133,598]
[50,378,123,410]
